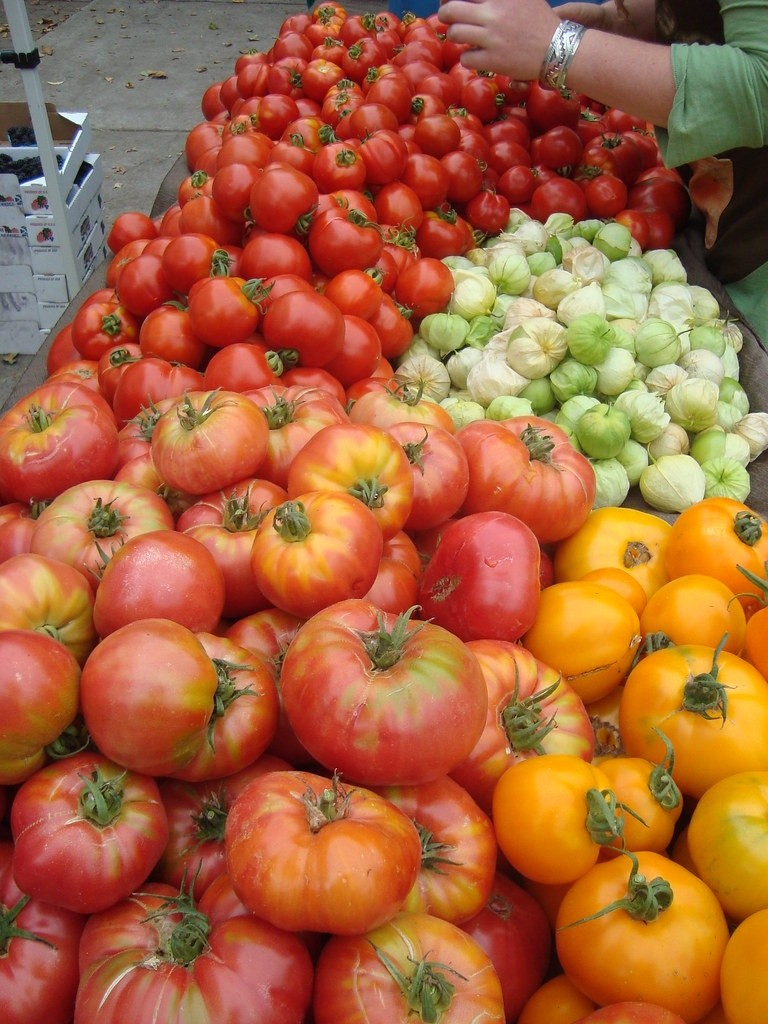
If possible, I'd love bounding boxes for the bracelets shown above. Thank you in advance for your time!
[538,18,589,98]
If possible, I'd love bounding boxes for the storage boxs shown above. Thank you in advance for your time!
[0,102,108,354]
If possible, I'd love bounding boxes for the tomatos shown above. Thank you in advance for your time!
[0,381,768,1024]
[46,0,690,406]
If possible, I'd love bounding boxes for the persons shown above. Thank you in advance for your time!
[437,0,768,353]
